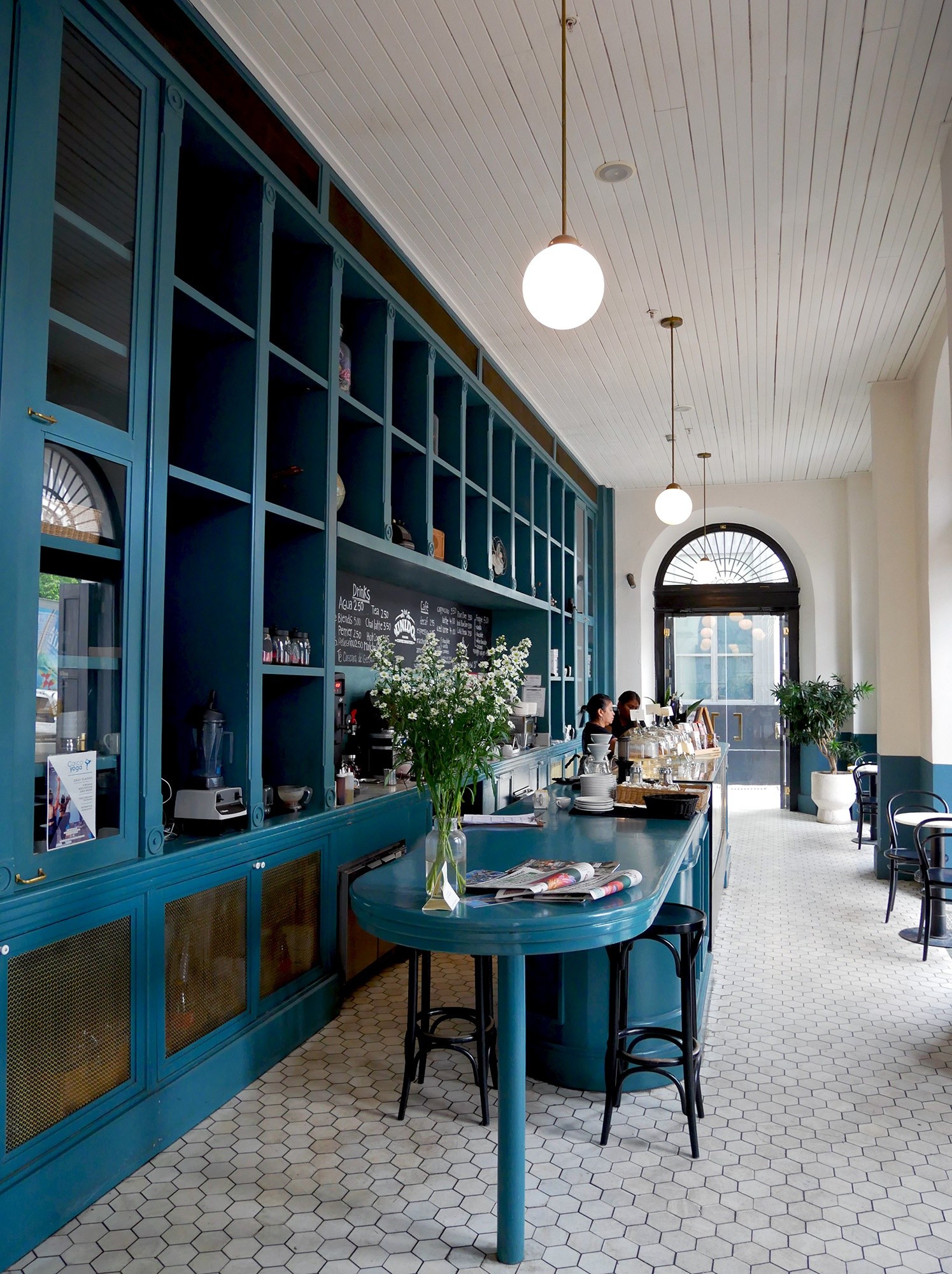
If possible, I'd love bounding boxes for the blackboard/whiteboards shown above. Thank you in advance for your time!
[335,568,494,673]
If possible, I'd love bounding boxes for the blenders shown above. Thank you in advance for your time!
[171,688,248,833]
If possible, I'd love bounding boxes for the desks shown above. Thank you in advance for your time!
[894,812,952,947]
[847,765,877,845]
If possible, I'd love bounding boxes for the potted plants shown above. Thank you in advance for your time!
[770,672,876,824]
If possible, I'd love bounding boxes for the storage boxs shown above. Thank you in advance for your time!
[550,649,559,676]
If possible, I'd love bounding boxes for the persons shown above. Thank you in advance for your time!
[49,776,70,847]
[578,691,646,775]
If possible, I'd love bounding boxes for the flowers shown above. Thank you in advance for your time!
[367,629,532,895]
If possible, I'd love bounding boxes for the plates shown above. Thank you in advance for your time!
[574,773,617,814]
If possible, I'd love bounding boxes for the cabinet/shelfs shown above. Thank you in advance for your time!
[0,0,618,1271]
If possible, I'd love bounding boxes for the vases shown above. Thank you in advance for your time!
[425,815,466,898]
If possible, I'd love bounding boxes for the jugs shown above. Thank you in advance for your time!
[583,750,612,774]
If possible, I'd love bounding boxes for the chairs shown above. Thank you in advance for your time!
[853,753,877,850]
[884,790,952,961]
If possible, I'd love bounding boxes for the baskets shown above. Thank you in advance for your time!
[616,783,711,810]
[643,794,699,819]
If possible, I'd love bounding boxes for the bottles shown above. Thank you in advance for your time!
[336,767,354,806]
[338,324,351,394]
[263,626,311,667]
[629,766,674,787]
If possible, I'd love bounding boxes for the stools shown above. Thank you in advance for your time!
[397,947,498,1126]
[600,903,708,1158]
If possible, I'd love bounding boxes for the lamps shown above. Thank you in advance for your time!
[693,453,717,583]
[654,316,693,526]
[523,0,606,329]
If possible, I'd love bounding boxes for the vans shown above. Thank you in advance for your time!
[35,689,58,755]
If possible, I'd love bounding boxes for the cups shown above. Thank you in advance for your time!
[104,733,120,755]
[501,744,513,757]
[277,785,313,809]
[383,769,402,785]
[586,743,610,761]
[556,797,571,810]
[590,733,613,744]
